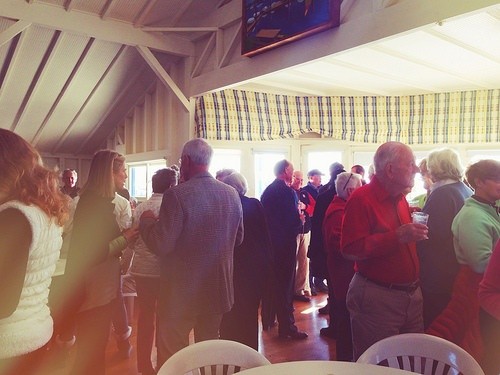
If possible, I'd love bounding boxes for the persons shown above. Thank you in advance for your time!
[140,138,245,375]
[215,167,268,374]
[0,128,81,375]
[427,158,500,375]
[132,168,179,375]
[315,162,367,294]
[415,148,474,327]
[341,142,430,367]
[62,149,140,375]
[289,168,326,301]
[112,186,142,326]
[322,173,363,362]
[260,159,313,339]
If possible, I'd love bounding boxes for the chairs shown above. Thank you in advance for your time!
[156,340,271,375]
[356,333,485,375]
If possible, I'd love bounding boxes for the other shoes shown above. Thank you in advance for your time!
[294,294,311,302]
[320,323,337,339]
[318,298,330,314]
[278,331,309,340]
[310,282,329,296]
[262,321,279,331]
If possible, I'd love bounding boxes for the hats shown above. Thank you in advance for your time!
[306,169,325,176]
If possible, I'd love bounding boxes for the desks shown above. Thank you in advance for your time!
[231,361,422,375]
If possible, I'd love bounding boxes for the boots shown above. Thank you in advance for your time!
[111,325,132,359]
[53,334,76,368]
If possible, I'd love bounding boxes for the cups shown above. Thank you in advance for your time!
[411,212,429,226]
[410,200,419,217]
[299,211,304,225]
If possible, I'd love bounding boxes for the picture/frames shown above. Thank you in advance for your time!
[239,0,340,57]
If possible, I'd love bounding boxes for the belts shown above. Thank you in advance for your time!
[360,272,421,293]
